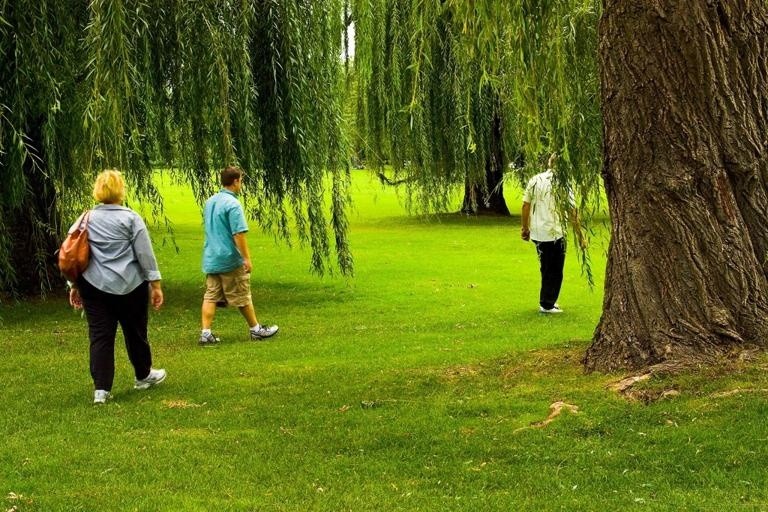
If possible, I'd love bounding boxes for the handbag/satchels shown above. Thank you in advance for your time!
[59,228,91,282]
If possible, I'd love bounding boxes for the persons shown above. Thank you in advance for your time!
[198,169,279,343]
[65,169,167,404]
[520,150,586,315]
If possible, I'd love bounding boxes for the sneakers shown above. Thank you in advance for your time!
[198,332,221,346]
[93,390,114,405]
[541,303,562,313]
[134,367,166,390]
[250,324,279,341]
